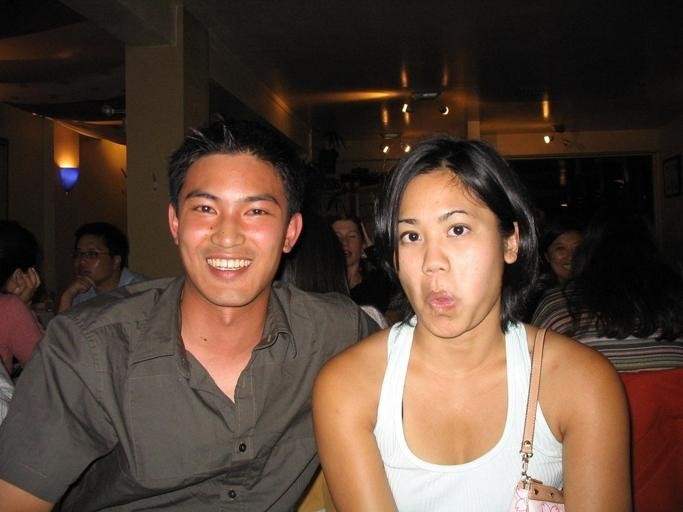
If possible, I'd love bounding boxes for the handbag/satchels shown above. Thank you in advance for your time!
[510,473,567,512]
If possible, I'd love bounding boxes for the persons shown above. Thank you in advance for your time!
[522,222,680,374]
[54,223,151,314]
[312,131,637,511]
[0,111,384,512]
[280,210,388,315]
[0,223,43,378]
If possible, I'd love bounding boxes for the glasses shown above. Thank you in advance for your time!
[70,248,117,261]
